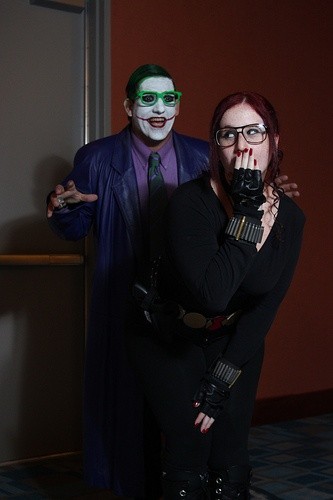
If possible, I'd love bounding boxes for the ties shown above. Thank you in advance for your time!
[146,152,170,261]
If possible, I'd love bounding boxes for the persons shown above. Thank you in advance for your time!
[46,64,300,500]
[150,92,306,500]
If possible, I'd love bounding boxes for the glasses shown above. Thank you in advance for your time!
[135,89,183,107]
[214,123,269,148]
